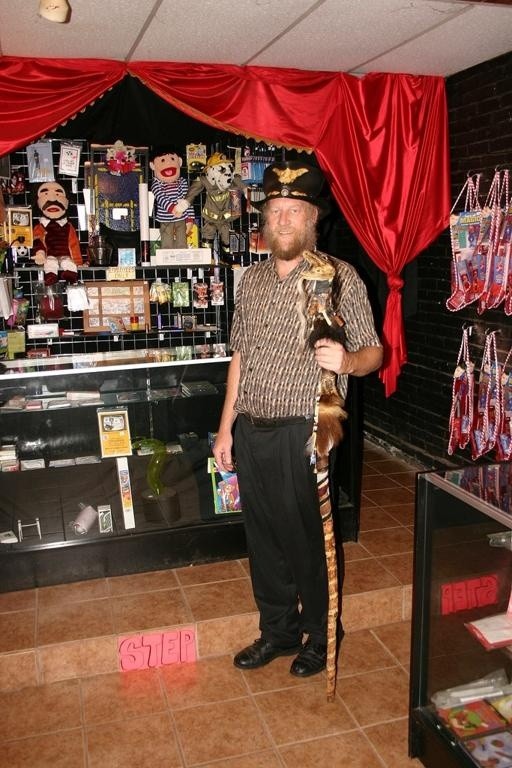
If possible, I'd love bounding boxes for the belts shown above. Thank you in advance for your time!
[246,416,306,428]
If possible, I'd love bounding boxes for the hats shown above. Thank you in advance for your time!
[248,161,333,209]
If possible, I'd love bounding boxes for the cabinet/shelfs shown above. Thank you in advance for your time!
[408,459,512,768]
[0,351,364,595]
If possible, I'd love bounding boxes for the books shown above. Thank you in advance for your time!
[438,698,507,737]
[0,379,219,412]
[459,730,512,766]
[210,458,241,513]
[0,444,21,472]
[97,409,132,457]
[484,692,512,729]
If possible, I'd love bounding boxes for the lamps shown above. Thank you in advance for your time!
[38,0,71,24]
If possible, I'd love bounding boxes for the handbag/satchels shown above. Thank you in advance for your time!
[448,329,512,461]
[446,170,512,317]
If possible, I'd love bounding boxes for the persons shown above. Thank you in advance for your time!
[208,162,387,680]
[174,150,249,256]
[31,181,85,286]
[149,145,194,247]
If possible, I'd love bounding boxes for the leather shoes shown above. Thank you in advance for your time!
[234,631,345,677]
